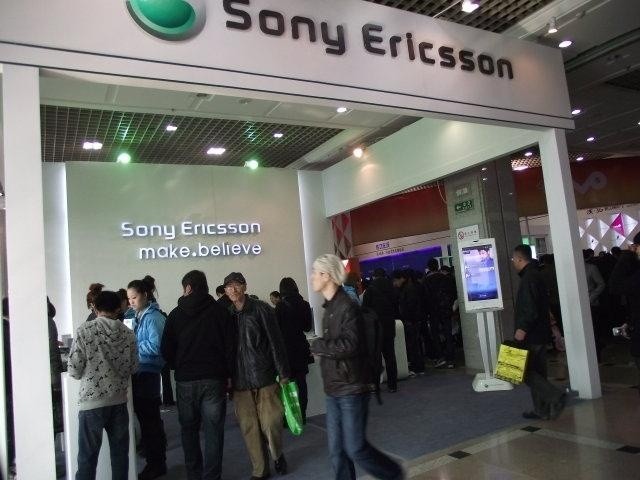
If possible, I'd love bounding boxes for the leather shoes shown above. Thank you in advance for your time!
[549,399,566,421]
[522,410,549,420]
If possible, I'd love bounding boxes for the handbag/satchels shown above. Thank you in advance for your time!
[280,380,305,437]
[494,343,530,387]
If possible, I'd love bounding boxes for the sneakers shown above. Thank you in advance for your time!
[250,472,271,480]
[445,362,456,369]
[369,387,378,394]
[409,370,417,378]
[387,387,397,393]
[434,360,447,369]
[274,453,288,475]
[417,371,425,377]
[138,462,168,480]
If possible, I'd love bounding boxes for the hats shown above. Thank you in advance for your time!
[223,272,246,287]
[313,253,349,287]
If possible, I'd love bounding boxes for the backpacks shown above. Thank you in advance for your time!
[358,308,384,384]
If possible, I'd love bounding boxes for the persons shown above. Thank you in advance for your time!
[47,296,64,477]
[542,231,640,348]
[512,243,570,421]
[356,259,456,395]
[68,271,313,480]
[2,297,16,477]
[478,249,493,267]
[309,254,405,479]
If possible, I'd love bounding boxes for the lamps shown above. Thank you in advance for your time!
[548,16,559,33]
